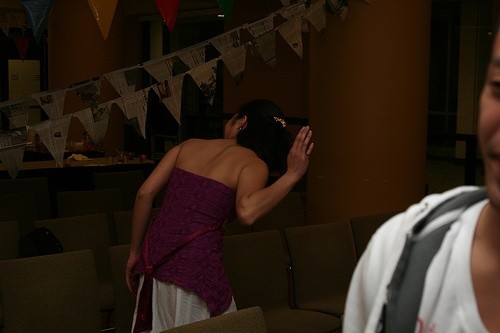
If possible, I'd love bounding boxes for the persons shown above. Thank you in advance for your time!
[124,99,315,333]
[342,30,500,333]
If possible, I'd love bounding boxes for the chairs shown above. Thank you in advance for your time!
[0,171,402,333]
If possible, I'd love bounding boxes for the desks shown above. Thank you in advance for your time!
[0,153,154,219]
